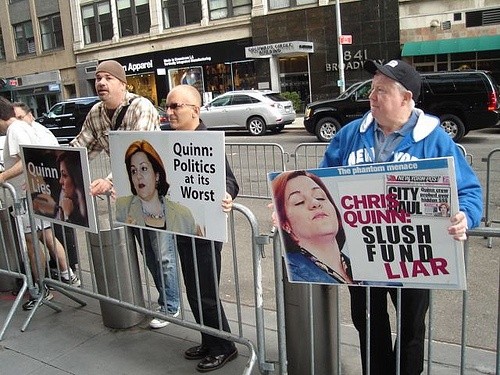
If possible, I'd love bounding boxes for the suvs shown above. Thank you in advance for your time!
[304,67,500,143]
[34,96,102,142]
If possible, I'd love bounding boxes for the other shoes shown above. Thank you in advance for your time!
[12,290,19,296]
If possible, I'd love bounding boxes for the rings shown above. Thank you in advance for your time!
[465,227,468,233]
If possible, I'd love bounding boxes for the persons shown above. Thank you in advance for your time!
[54,151,89,228]
[0,97,81,310]
[433,203,451,217]
[167,85,239,372]
[267,59,482,375]
[69,60,180,328]
[271,171,403,286]
[116,140,203,237]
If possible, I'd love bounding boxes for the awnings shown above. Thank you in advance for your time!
[402,35,500,56]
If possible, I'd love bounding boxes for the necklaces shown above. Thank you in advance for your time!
[142,207,165,220]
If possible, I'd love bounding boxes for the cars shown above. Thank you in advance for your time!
[198,90,297,137]
[335,81,369,100]
[153,104,177,132]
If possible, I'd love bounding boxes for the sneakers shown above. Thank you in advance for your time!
[149,307,178,328]
[45,274,81,291]
[22,286,53,310]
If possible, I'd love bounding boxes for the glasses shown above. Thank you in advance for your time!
[18,113,27,121]
[165,103,197,111]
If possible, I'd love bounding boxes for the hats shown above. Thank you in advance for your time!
[364,58,421,102]
[96,60,126,84]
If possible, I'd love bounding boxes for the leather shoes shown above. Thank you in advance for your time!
[197,342,238,371]
[184,344,210,357]
